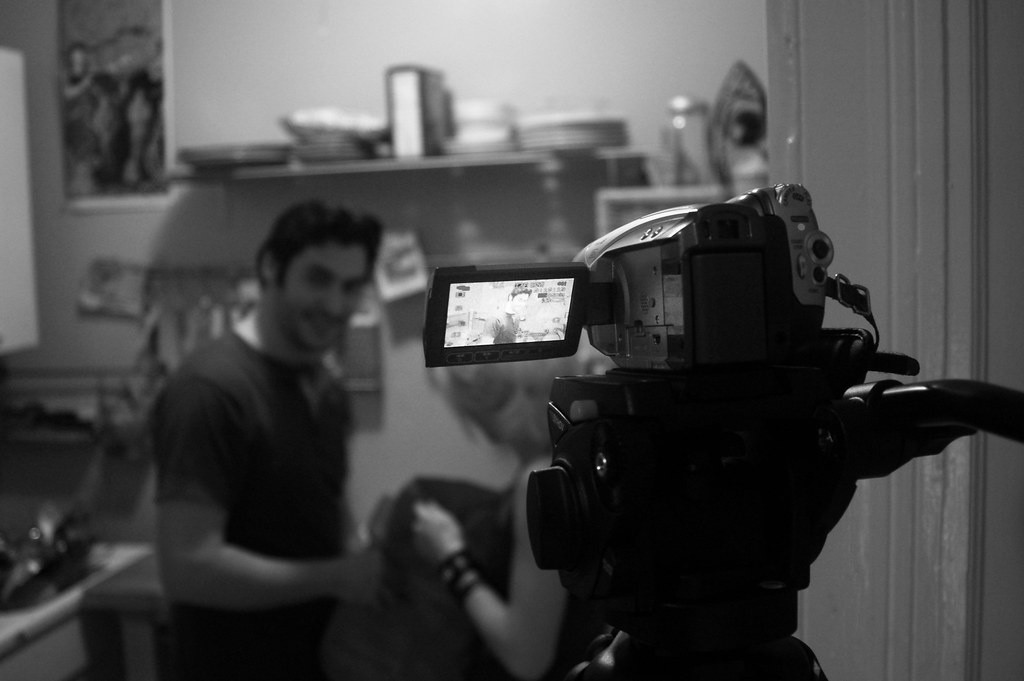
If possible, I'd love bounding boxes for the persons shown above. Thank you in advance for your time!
[480,287,531,345]
[60,33,163,198]
[416,355,603,681]
[149,196,388,681]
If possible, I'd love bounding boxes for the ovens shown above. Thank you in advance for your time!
[597,189,727,242]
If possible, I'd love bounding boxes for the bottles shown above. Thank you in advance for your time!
[665,96,711,187]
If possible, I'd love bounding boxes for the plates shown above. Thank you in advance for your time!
[281,105,390,162]
[440,110,628,158]
[174,144,294,171]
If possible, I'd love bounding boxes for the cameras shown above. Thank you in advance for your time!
[422,183,835,369]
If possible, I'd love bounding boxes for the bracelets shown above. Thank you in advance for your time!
[435,550,479,606]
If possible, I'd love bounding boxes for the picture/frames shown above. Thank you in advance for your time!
[52,0,175,214]
